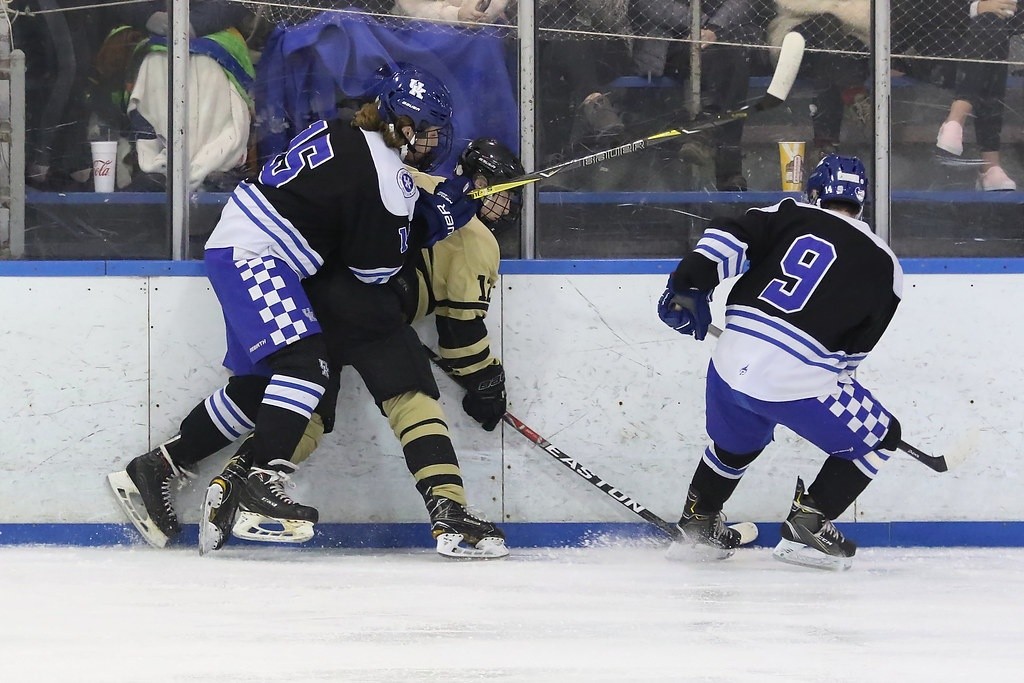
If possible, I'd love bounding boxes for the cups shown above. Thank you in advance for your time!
[778,142,805,192]
[90,141,118,192]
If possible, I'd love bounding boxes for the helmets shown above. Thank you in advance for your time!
[376,64,456,173]
[808,155,869,210]
[452,137,525,236]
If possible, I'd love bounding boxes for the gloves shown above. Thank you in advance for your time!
[431,176,481,238]
[462,387,507,430]
[658,278,712,341]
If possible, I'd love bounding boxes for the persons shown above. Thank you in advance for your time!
[108,63,485,551]
[892,0,1024,193]
[658,157,903,571]
[765,0,871,159]
[13,0,773,191]
[198,137,528,558]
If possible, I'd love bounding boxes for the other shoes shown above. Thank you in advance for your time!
[586,97,626,147]
[541,152,581,193]
[806,145,837,167]
[839,85,871,129]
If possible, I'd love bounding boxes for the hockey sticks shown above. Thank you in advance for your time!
[418,343,759,549]
[670,302,957,474]
[460,31,806,203]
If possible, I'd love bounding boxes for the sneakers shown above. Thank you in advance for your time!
[668,487,741,561]
[680,139,716,168]
[774,477,856,569]
[976,166,1015,190]
[108,436,178,548]
[716,173,748,192]
[232,458,319,542]
[937,121,964,157]
[422,487,509,555]
[199,452,254,557]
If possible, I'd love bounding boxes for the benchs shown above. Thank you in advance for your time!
[24,72,1024,204]
